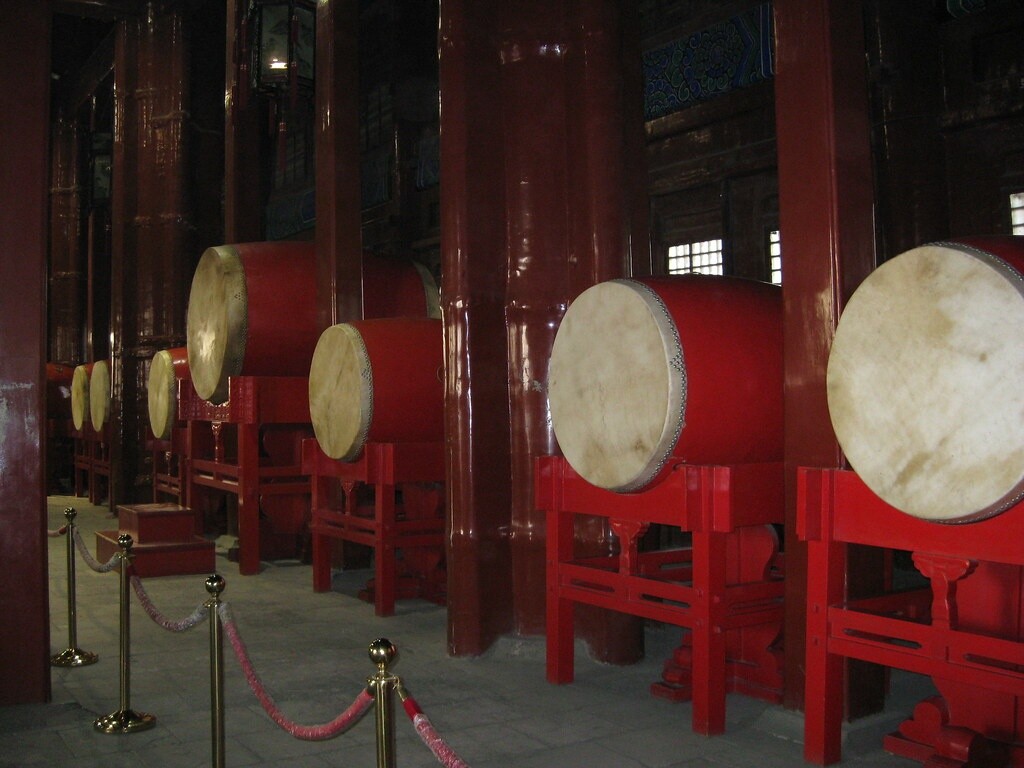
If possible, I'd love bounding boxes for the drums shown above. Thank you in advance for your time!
[71,363,93,432]
[186,238,440,404]
[88,359,111,433]
[548,275,786,494]
[148,347,192,439]
[308,317,444,465]
[826,237,1024,527]
[46,362,73,406]
